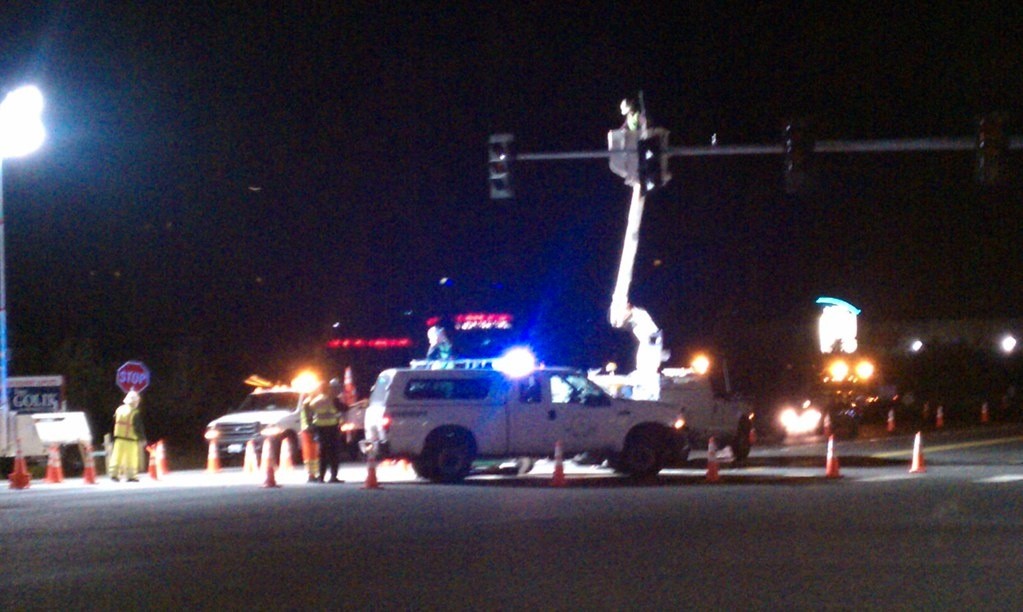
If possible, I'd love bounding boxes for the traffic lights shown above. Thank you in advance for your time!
[980,115,1007,188]
[786,123,813,190]
[488,134,515,198]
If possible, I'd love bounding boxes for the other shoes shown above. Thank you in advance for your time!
[126,479,138,482]
[111,476,119,482]
[328,478,343,484]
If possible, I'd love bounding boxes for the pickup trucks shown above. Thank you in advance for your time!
[362,366,689,485]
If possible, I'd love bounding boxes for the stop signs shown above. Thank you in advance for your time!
[115,362,149,392]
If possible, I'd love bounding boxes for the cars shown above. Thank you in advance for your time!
[205,389,307,465]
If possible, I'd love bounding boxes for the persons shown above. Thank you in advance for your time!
[617,98,648,132]
[427,327,453,369]
[300,379,369,484]
[108,391,147,482]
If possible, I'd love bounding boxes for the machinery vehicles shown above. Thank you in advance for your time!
[585,130,757,462]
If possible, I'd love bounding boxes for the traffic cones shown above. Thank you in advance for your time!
[886,401,989,432]
[702,439,722,482]
[8,436,31,488]
[80,438,172,486]
[258,458,280,488]
[204,441,222,471]
[343,366,358,408]
[547,440,570,485]
[40,446,65,482]
[242,437,298,473]
[822,435,845,478]
[907,432,928,474]
[357,452,385,490]
